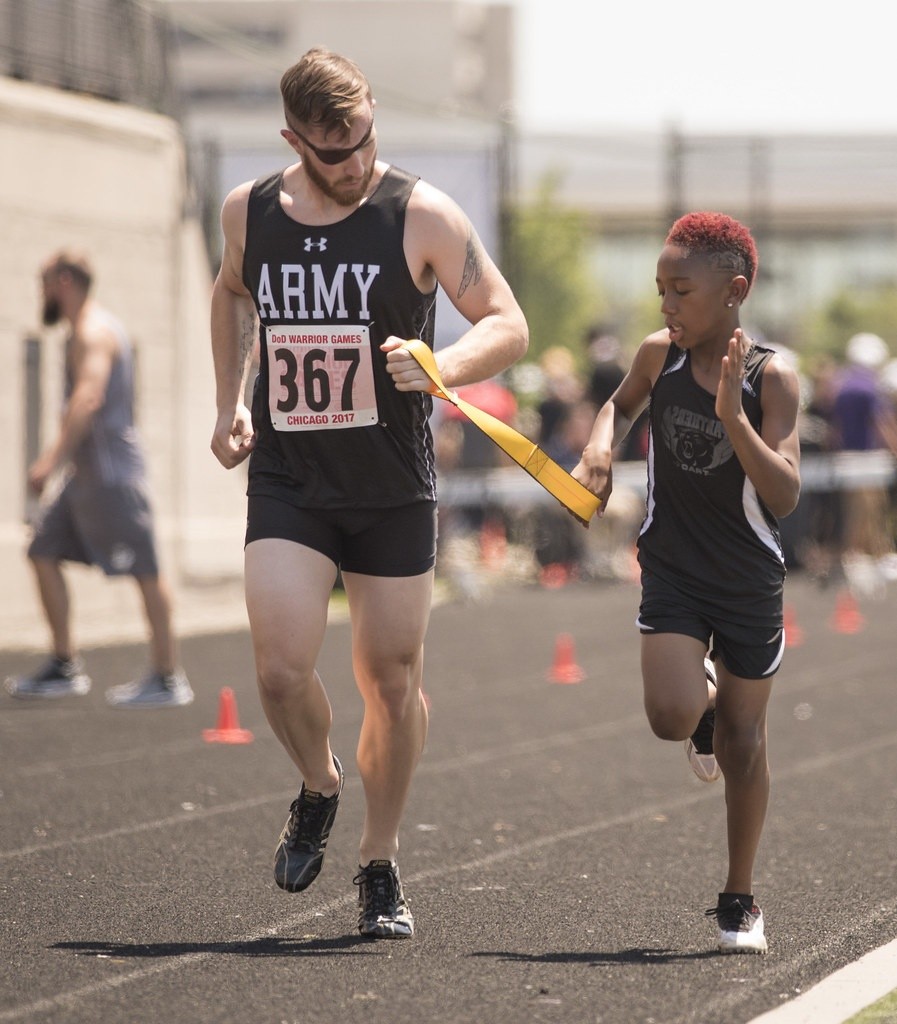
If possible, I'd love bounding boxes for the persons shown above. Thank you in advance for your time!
[209,47,530,940]
[560,210,802,955]
[427,324,897,593]
[4,248,198,710]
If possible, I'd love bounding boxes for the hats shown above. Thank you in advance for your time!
[844,332,889,368]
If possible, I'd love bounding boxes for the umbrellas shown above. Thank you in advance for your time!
[440,381,516,423]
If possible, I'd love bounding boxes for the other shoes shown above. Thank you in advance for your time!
[4,653,91,699]
[104,665,195,709]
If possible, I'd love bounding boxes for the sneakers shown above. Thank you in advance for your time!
[352,860,414,938]
[684,657,721,784]
[272,754,345,893]
[704,892,768,956]
